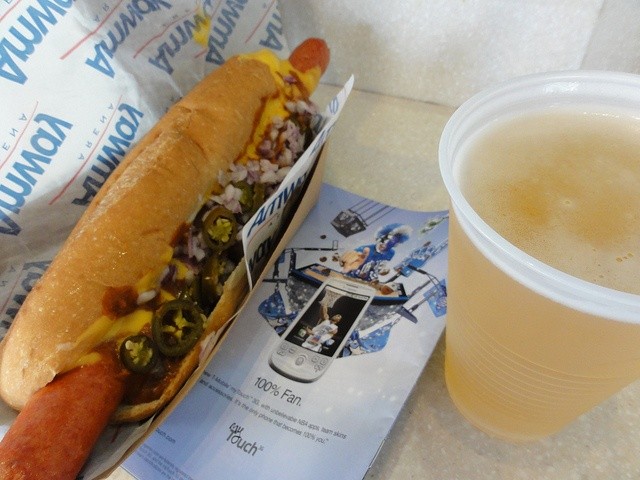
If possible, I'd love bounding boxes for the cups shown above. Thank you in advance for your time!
[436,70,640,445]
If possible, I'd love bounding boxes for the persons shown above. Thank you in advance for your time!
[298,301,343,353]
[341,221,413,275]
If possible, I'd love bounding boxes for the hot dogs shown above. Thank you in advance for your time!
[1,37,356,479]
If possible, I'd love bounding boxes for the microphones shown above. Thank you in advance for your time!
[407,264,427,275]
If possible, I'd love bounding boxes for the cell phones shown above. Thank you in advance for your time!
[266,276,376,384]
